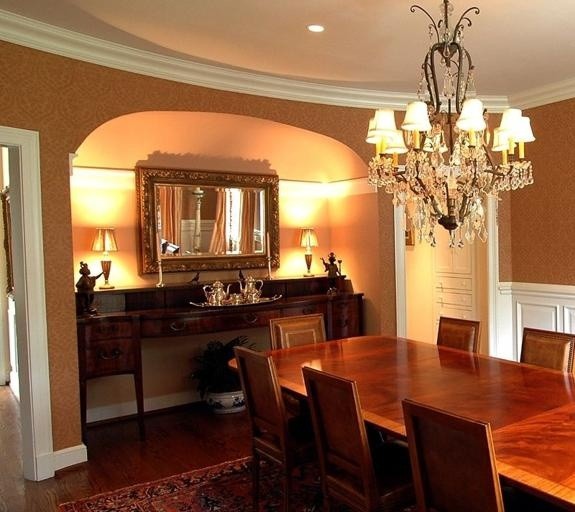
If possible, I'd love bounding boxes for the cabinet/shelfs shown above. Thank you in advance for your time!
[74,270,365,444]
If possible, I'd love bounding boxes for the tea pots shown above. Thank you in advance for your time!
[237,276,263,303]
[200,280,228,305]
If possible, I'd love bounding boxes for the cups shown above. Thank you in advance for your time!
[229,293,242,303]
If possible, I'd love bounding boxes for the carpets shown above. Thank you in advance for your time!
[55,451,415,512]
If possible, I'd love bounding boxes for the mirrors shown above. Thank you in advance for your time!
[132,164,280,276]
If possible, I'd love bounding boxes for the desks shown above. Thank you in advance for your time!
[226,332,575,511]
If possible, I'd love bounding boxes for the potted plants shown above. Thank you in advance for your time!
[186,333,265,416]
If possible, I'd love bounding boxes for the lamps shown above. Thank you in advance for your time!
[300,228,319,277]
[89,228,118,290]
[363,0,537,250]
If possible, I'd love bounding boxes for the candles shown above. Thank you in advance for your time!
[155,232,162,261]
[266,229,271,257]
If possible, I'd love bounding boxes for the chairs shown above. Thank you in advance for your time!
[518,326,575,377]
[268,309,328,351]
[396,395,523,511]
[231,340,317,510]
[299,365,418,512]
[436,314,480,357]
